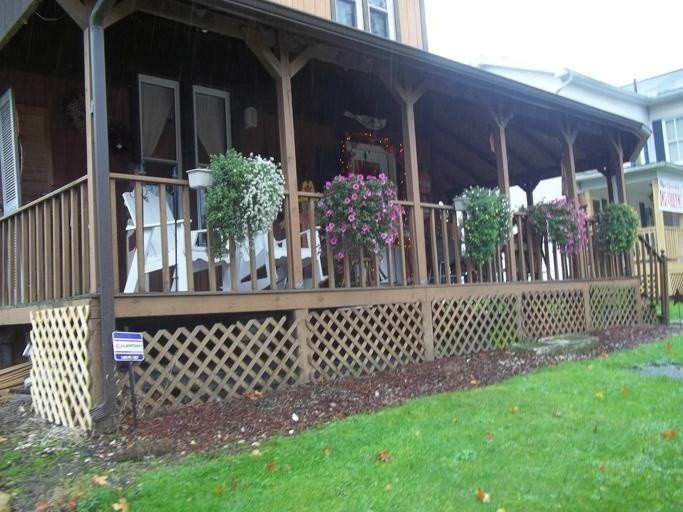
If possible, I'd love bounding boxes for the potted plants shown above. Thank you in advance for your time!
[453,185,512,267]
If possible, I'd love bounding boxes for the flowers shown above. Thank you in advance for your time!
[528,196,588,256]
[206,149,286,260]
[337,131,406,206]
[318,173,406,259]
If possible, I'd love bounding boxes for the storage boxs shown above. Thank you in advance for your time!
[186,169,213,189]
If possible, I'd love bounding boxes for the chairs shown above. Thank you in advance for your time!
[223,226,330,291]
[122,185,231,293]
